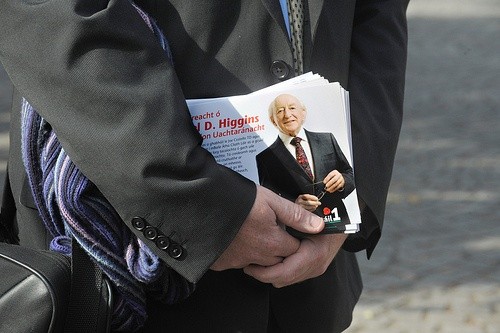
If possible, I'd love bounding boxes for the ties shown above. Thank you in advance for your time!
[286,0,307,77]
[290,137,313,180]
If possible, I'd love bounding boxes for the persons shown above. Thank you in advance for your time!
[254,94,357,228]
[0,1,407,333]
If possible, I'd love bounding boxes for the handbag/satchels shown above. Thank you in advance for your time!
[0,241,112,333]
